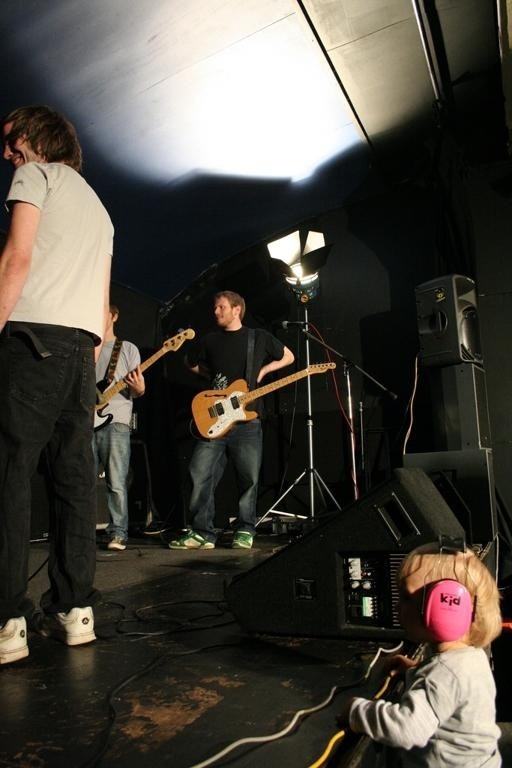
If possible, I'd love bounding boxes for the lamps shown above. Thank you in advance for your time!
[255,226,345,541]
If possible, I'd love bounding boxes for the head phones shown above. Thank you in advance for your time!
[419,532,477,644]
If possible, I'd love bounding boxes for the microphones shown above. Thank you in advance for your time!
[282,320,306,330]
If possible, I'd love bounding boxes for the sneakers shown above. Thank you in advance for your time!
[107,534,128,551]
[169,529,216,550]
[0,614,30,667]
[34,605,97,647]
[231,529,254,550]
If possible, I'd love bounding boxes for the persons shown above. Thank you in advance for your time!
[168,291,296,550]
[335,540,503,768]
[1,104,115,671]
[95,301,146,552]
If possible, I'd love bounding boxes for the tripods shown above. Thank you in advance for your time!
[253,307,342,530]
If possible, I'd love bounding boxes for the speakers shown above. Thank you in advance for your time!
[414,274,485,370]
[222,465,467,643]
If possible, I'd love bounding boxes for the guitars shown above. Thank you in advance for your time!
[192,361,336,439]
[94,328,195,432]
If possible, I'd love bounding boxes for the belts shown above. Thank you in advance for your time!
[4,322,54,358]
